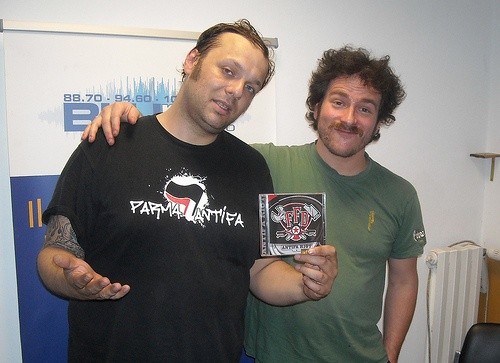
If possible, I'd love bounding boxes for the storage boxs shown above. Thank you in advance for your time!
[259,192,328,256]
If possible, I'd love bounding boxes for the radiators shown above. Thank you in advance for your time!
[424,246,487,363]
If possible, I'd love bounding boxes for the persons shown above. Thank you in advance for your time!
[80,44,426,363]
[37,19,340,363]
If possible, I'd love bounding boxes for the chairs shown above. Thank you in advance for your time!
[453,323,500,363]
